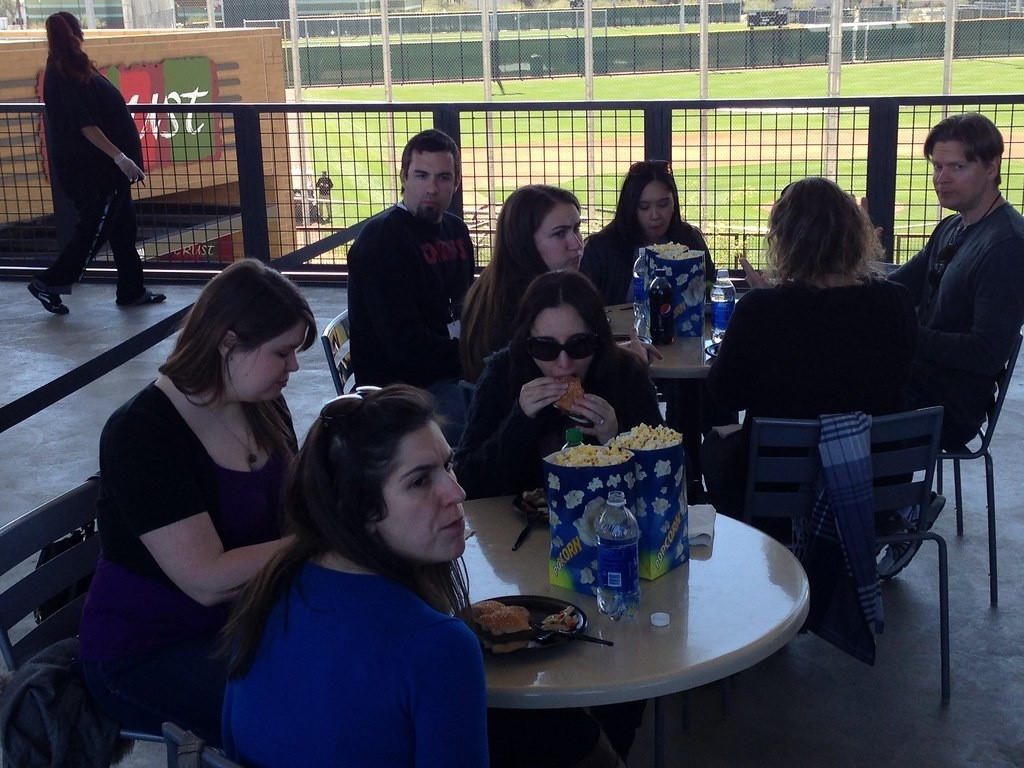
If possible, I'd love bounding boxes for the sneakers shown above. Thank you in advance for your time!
[877,491,946,581]
[117,293,166,305]
[28,282,69,314]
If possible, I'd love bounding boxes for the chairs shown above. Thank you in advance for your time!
[743,403,952,703]
[911,327,1024,609]
[0,471,101,675]
[320,308,358,399]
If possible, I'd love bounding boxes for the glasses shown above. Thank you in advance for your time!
[628,160,673,180]
[526,333,598,362]
[319,385,382,453]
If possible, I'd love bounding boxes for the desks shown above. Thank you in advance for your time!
[413,494,811,767]
[605,293,738,500]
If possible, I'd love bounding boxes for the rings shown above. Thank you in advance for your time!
[597,417,604,425]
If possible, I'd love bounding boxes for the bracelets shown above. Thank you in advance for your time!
[114,153,124,165]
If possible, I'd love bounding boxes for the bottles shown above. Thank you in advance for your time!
[709,270,736,342]
[633,248,648,317]
[596,491,642,620]
[560,428,586,452]
[648,269,674,345]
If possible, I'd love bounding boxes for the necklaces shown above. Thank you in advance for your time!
[977,192,1001,222]
[198,395,258,464]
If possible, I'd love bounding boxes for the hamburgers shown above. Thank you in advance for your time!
[555,375,586,415]
[461,599,534,655]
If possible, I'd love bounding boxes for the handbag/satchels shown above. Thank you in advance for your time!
[32,470,101,623]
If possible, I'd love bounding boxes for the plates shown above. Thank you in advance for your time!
[452,595,588,658]
[613,334,652,348]
[510,491,550,520]
[704,343,719,358]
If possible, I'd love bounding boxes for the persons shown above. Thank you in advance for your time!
[345,129,477,439]
[580,160,740,503]
[453,269,664,500]
[850,112,1024,579]
[75,258,318,747]
[219,384,490,768]
[316,170,334,223]
[699,178,922,540]
[26,11,166,315]
[459,184,664,434]
